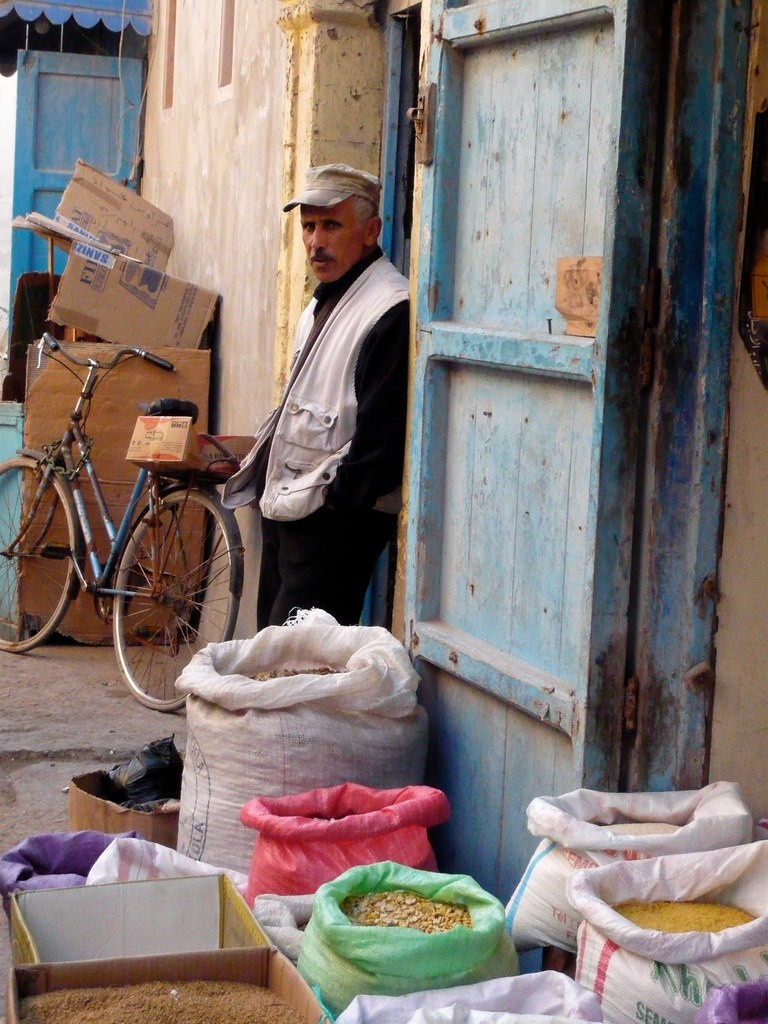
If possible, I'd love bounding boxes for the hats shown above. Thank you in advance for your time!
[282,163,382,214]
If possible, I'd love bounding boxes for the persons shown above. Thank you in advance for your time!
[219,167,410,631]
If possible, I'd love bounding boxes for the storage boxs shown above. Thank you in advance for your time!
[126,417,262,474]
[1,272,110,403]
[6,874,332,1024]
[69,762,185,851]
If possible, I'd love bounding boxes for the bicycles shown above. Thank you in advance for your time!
[0,334,249,713]
